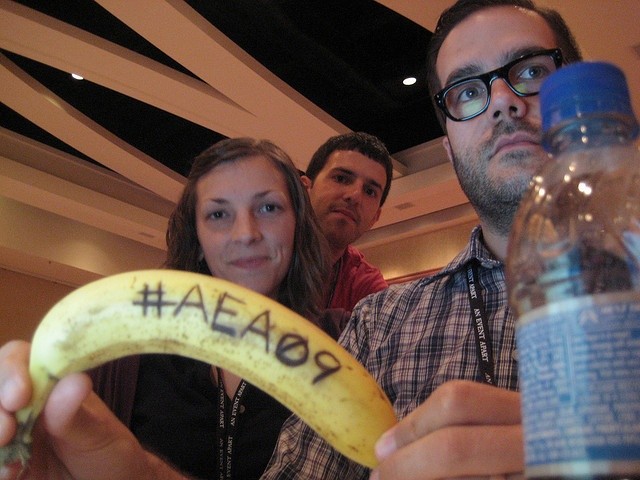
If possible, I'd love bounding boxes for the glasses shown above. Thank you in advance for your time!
[432,48,570,122]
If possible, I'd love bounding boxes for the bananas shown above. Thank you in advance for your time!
[0,266,399,469]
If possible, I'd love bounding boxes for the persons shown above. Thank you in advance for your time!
[85,138,350,480]
[258,4,640,479]
[299,132,397,342]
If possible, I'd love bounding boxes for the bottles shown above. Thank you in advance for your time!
[503,60,640,480]
